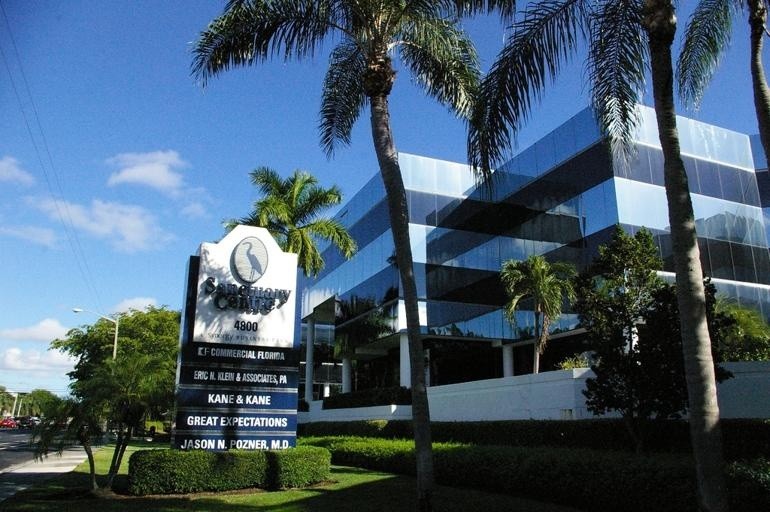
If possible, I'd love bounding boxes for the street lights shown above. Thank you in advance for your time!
[71,307,122,375]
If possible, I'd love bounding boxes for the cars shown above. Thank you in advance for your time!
[0,416,41,430]
